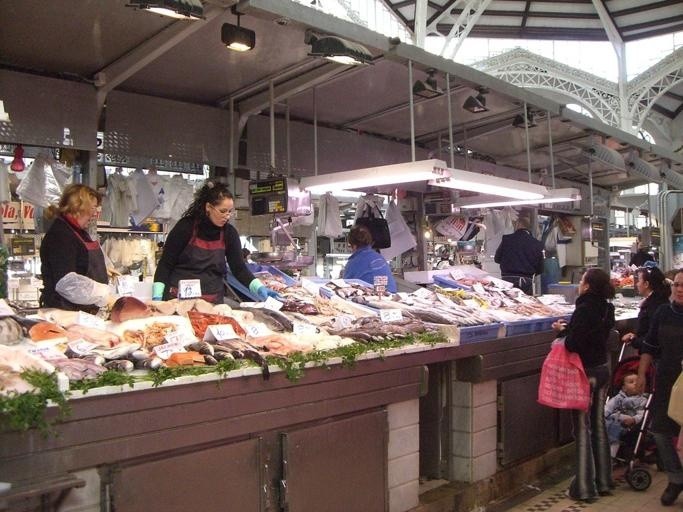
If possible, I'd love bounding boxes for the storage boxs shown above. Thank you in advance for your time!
[463,301,552,336]
[432,271,501,291]
[519,293,579,327]
[303,278,392,313]
[222,263,300,303]
[376,307,501,345]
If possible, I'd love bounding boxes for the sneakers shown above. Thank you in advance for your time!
[660,480,683,506]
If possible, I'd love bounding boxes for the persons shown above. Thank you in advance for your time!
[551,269,615,502]
[621,267,671,355]
[39,183,121,314]
[343,226,398,294]
[629,241,654,267]
[604,373,648,464]
[636,268,683,506]
[493,217,544,295]
[152,184,282,307]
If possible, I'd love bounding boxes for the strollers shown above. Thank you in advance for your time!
[603,338,658,491]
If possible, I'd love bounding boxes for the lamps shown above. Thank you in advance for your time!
[125,1,206,23]
[463,90,490,116]
[582,136,627,175]
[221,14,256,54]
[512,115,536,130]
[412,68,444,101]
[301,58,448,195]
[307,35,375,68]
[448,81,583,211]
[428,74,548,198]
[666,168,683,190]
[628,156,664,183]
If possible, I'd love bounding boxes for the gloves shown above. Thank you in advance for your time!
[152,282,165,302]
[249,277,279,304]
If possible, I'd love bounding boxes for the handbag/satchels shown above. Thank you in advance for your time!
[537,337,591,413]
[357,202,391,248]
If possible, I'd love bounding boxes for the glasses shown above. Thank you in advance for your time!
[671,282,683,289]
[210,205,231,219]
